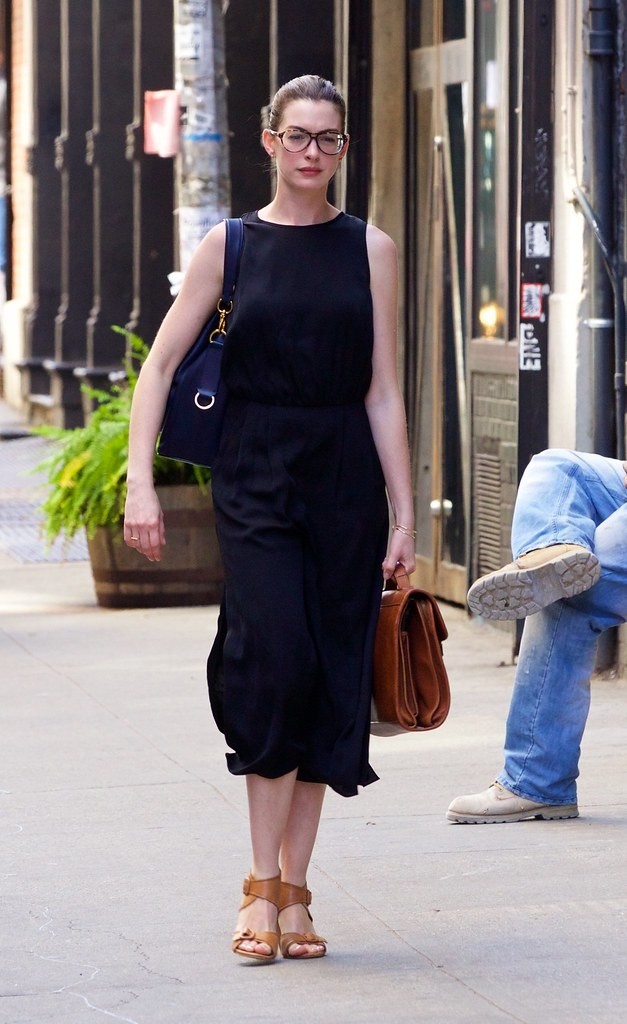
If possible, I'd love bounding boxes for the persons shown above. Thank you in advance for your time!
[123,75,416,961]
[444,448,627,824]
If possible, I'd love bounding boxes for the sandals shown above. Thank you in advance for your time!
[233,868,282,962]
[277,881,328,959]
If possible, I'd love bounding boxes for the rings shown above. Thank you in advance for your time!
[130,536,138,542]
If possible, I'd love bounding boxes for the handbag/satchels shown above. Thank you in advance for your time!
[372,562,451,731]
[156,218,244,470]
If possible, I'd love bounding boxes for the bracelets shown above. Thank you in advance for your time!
[392,524,417,539]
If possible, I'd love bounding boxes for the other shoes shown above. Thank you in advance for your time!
[466,544,601,621]
[445,780,579,824]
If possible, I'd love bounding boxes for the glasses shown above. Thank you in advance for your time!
[269,130,348,155]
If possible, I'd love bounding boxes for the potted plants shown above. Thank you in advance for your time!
[22,326,225,610]
